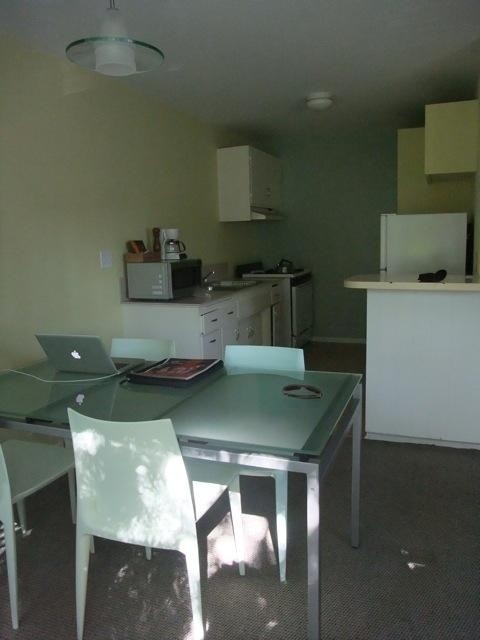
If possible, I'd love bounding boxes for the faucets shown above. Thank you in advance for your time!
[203,270,216,279]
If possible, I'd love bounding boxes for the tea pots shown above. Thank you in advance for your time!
[274,258,293,273]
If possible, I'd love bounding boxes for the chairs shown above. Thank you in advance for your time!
[68,407,245,640]
[0,439,76,631]
[224,344,304,581]
[111,337,177,367]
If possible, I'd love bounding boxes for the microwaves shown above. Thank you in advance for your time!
[126,257,202,300]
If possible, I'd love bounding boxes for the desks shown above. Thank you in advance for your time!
[0,356,369,638]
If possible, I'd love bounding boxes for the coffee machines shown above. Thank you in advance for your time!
[159,226,189,260]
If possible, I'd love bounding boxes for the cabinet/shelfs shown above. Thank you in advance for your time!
[238,288,261,345]
[396,99,479,224]
[121,303,222,361]
[262,282,282,346]
[217,145,283,222]
[222,299,240,362]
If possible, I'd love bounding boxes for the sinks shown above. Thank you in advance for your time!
[206,279,264,291]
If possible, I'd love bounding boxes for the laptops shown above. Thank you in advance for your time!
[30,333,143,377]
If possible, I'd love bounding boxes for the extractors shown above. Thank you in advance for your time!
[251,208,287,222]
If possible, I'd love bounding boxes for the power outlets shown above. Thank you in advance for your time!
[65,0,166,79]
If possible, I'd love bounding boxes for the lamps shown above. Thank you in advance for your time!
[304,90,335,112]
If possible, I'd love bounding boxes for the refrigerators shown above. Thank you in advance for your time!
[379,211,468,274]
